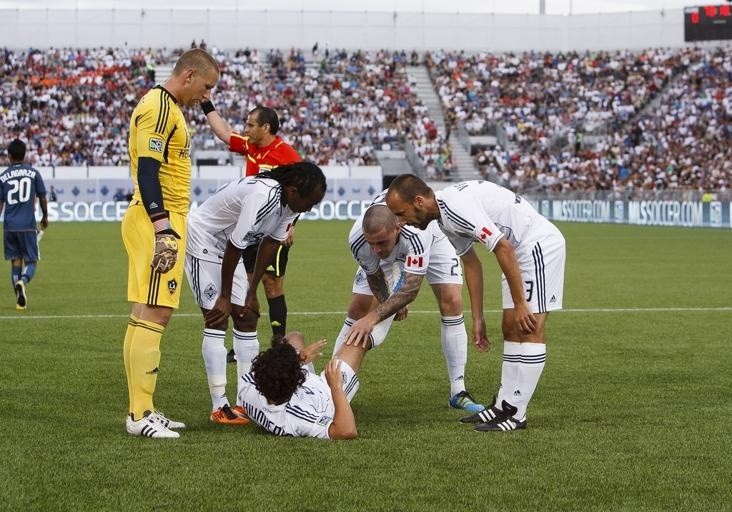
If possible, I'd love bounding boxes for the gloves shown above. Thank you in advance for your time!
[150,229,181,274]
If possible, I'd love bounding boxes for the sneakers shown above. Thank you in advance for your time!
[458,395,503,424]
[448,390,485,413]
[473,399,526,432]
[154,409,185,430]
[125,412,180,439]
[210,404,252,425]
[15,279,27,307]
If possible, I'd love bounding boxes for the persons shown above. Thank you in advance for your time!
[238,270,408,438]
[0,138,48,310]
[199,91,304,352]
[385,173,566,432]
[121,49,219,439]
[330,188,487,414]
[185,160,326,425]
[1,40,732,200]
